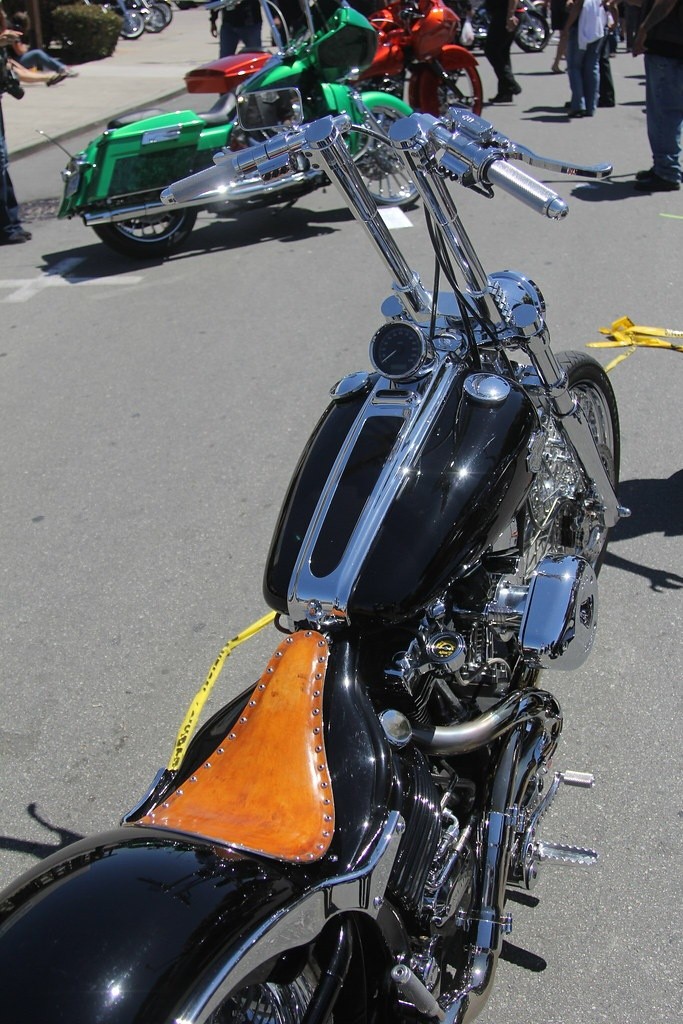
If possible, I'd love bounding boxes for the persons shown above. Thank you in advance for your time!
[482,0,522,103]
[631,0,683,189]
[209,0,392,59]
[0,9,79,245]
[547,0,639,117]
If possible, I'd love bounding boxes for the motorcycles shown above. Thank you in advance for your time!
[1,85,634,1024]
[33,0,553,263]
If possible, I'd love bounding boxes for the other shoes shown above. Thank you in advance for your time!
[489,95,513,106]
[17,228,31,240]
[47,73,66,87]
[596,96,615,108]
[509,84,521,94]
[551,66,565,74]
[637,170,658,180]
[566,106,586,116]
[59,69,78,77]
[635,174,680,190]
[587,109,595,116]
[0,235,25,244]
